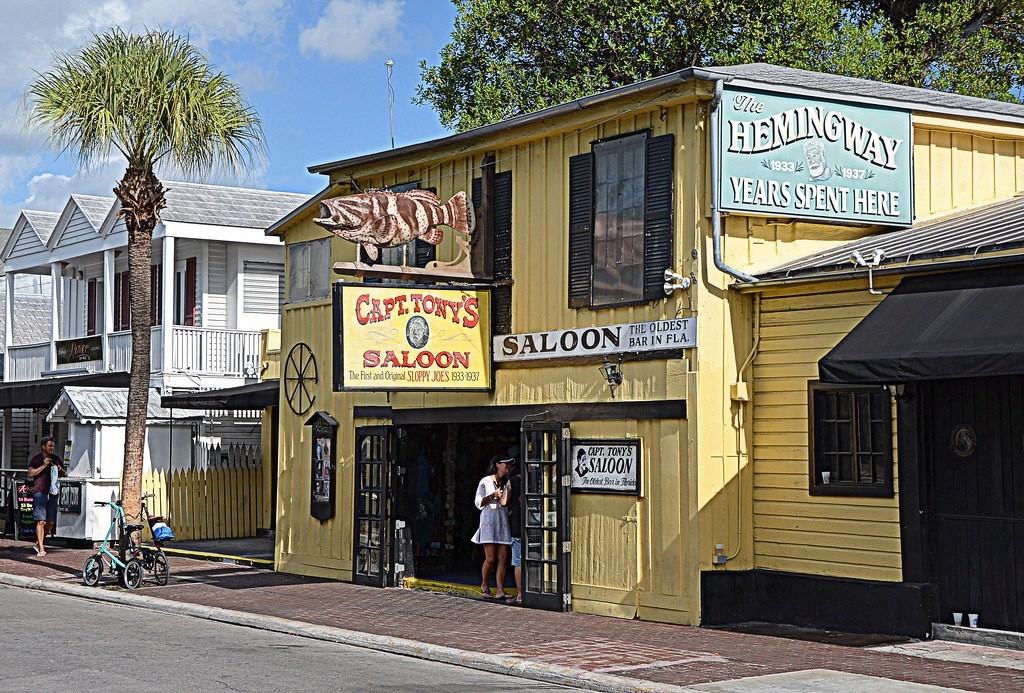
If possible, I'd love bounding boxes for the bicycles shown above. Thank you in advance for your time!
[140,492,170,586]
[80,500,145,590]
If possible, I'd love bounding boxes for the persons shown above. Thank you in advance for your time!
[509,474,521,604]
[27,437,67,556]
[470,454,518,595]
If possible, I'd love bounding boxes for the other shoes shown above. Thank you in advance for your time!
[506,596,523,606]
[33,543,46,556]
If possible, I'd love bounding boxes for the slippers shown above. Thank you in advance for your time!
[494,593,514,600]
[480,584,494,599]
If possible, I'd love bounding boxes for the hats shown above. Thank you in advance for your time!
[489,453,515,471]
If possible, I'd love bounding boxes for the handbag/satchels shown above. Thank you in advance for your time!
[48,463,61,495]
